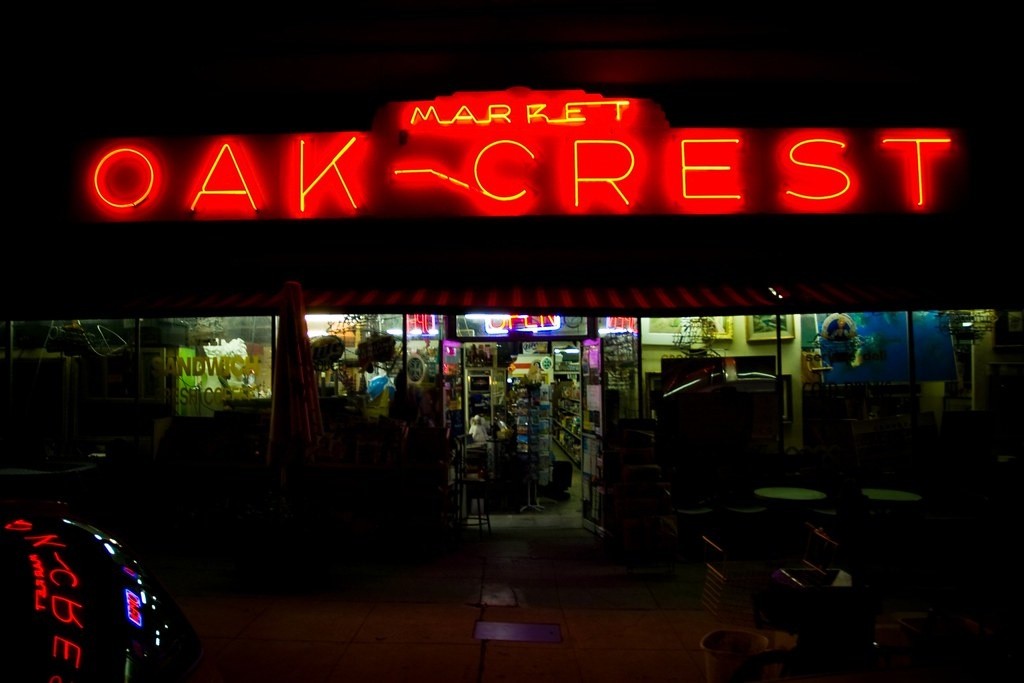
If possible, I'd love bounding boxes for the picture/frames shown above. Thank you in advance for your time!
[641,315,796,347]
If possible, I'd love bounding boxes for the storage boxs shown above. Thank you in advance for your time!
[699,629,769,683]
[603,420,677,582]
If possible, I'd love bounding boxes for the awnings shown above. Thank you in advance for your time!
[150,282,913,311]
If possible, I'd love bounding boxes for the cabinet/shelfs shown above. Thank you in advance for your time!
[463,363,582,513]
[0,319,191,518]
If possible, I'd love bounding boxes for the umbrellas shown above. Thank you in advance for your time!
[270,282,326,479]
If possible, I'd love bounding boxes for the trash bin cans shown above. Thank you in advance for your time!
[700,628,769,683]
